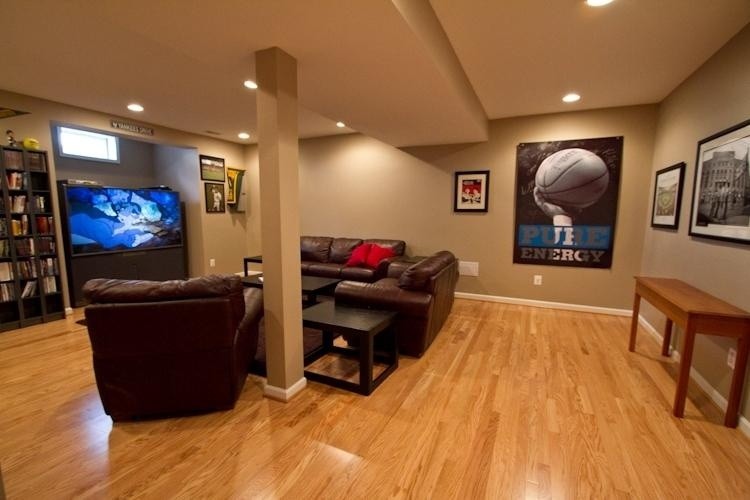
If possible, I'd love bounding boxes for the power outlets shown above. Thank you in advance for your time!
[726,347,737,370]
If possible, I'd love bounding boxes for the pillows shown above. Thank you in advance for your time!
[366,244,398,270]
[345,243,370,267]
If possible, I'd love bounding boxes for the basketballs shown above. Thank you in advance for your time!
[535,148,610,210]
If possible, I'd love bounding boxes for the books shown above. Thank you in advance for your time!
[0,149,60,302]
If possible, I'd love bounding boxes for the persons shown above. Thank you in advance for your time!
[462,188,473,204]
[532,186,584,262]
[6,128,16,147]
[471,188,481,205]
[209,184,223,211]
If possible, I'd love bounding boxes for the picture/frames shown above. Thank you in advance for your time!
[199,154,225,182]
[204,182,225,213]
[453,170,490,212]
[651,161,686,230]
[688,118,750,245]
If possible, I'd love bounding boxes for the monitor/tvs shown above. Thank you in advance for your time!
[62,184,184,257]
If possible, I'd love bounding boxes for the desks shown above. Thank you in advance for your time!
[628,276,750,428]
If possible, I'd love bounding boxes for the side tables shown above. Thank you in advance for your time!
[302,300,399,396]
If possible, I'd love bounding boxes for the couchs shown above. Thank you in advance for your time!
[300,236,406,283]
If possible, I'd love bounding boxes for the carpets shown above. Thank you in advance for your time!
[75,296,323,378]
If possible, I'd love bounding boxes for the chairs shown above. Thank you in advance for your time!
[82,274,263,423]
[334,250,459,358]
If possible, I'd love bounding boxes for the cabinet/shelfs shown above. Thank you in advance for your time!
[0,146,65,333]
[70,243,188,308]
